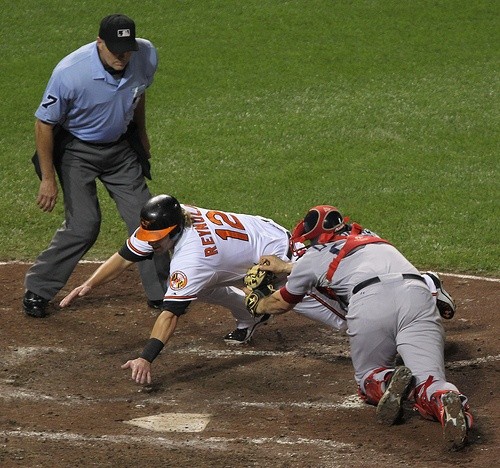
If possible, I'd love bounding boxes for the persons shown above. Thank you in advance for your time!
[58,193,348,384]
[22,13,187,318]
[242,205,474,455]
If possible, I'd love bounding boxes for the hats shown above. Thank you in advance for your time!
[99,14,140,53]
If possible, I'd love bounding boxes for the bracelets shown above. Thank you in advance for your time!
[139,338,165,363]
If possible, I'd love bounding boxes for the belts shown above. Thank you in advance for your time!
[352,274,426,295]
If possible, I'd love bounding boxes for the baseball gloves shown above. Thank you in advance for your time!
[243,263,277,317]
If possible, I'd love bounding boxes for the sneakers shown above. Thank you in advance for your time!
[426,270,456,320]
[376,366,412,426]
[146,299,162,309]
[442,390,468,452]
[22,288,50,316]
[224,313,273,347]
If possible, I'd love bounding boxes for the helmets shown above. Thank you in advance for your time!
[303,207,350,245]
[134,193,185,242]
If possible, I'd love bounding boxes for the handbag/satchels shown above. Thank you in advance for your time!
[126,121,152,182]
[31,123,75,181]
